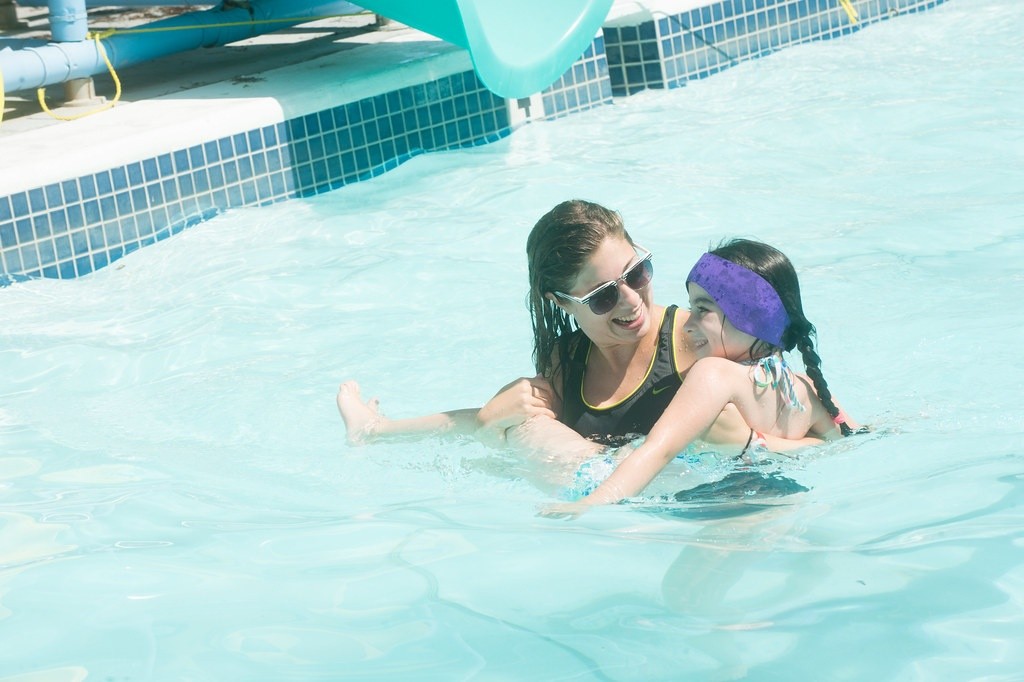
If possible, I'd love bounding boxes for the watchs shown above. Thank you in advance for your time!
[755,430,768,453]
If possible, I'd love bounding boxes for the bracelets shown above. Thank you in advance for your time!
[735,429,753,459]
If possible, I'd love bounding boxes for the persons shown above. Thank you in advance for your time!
[337,239,857,506]
[475,198,825,453]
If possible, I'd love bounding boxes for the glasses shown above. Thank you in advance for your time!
[552,240,653,316]
